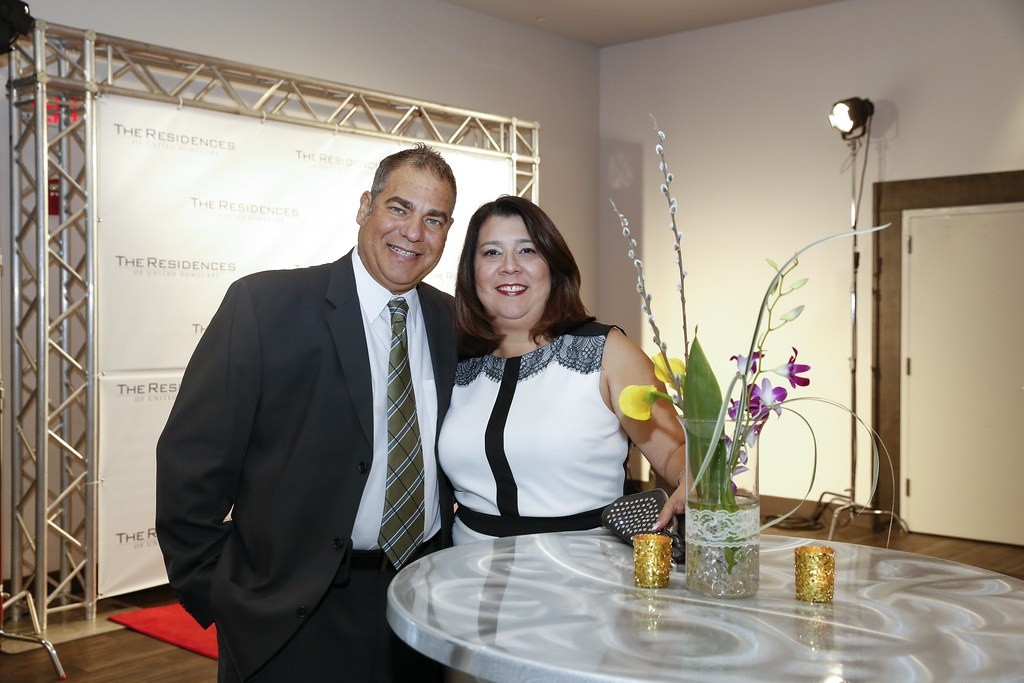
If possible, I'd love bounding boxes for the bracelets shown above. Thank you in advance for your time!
[676,469,685,487]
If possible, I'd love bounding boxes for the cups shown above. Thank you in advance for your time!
[794,545,834,603]
[633,533,672,588]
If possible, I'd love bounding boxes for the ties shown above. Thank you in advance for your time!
[376,300,426,573]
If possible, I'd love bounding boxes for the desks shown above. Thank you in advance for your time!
[385,530,1024,683]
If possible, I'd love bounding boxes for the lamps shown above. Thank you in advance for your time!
[809,96,910,543]
[0,0,35,56]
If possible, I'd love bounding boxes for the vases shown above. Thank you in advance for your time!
[675,413,759,599]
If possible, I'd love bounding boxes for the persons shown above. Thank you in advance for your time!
[157,148,496,683]
[436,195,704,545]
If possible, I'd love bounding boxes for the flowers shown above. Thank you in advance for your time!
[608,112,895,550]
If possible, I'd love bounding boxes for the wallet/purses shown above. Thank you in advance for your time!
[600,487,685,568]
